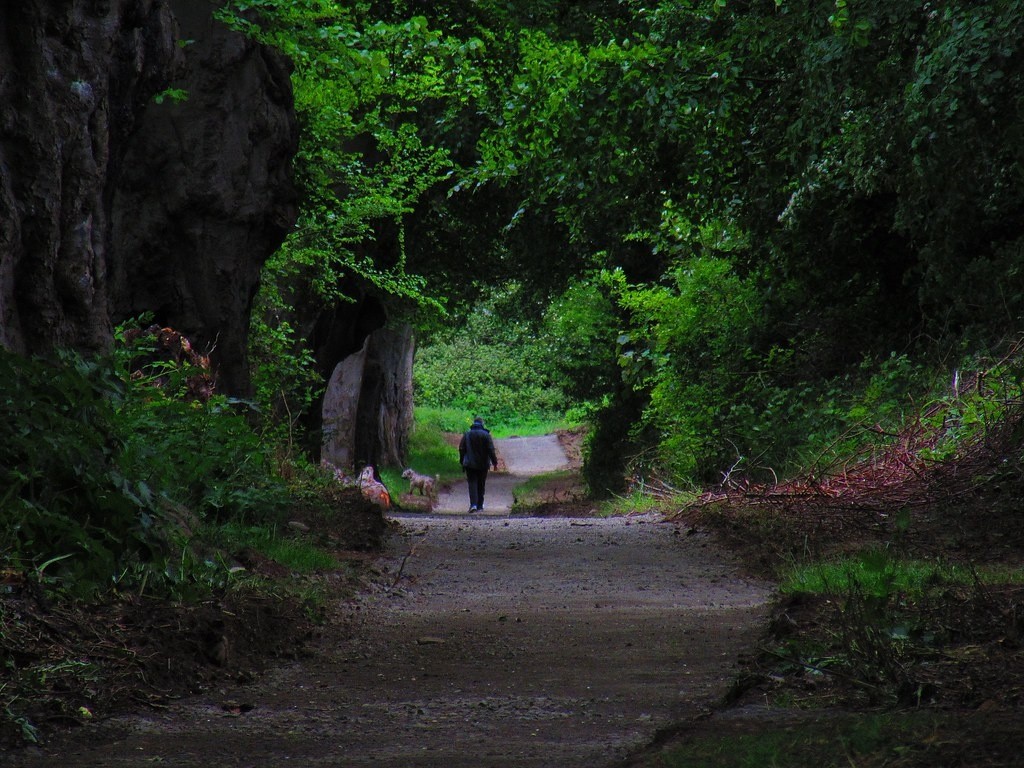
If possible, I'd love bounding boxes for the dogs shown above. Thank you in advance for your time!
[401,468,440,498]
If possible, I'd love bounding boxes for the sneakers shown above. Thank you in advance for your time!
[468,504,484,513]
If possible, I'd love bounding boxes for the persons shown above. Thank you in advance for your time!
[459,417,498,513]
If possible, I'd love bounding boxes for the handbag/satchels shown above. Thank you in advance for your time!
[462,454,492,476]
